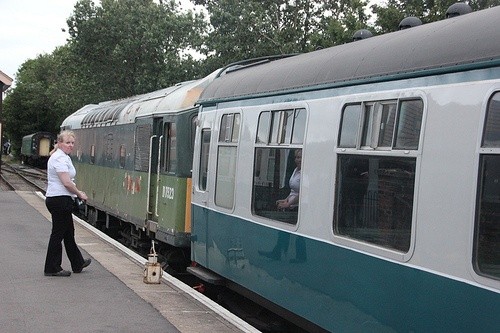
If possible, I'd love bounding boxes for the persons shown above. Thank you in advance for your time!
[276,148,302,211]
[43,128,91,277]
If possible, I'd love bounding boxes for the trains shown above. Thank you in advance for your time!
[20,131,53,169]
[58,1,500,333]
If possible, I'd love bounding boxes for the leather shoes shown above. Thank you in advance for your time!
[72,256,91,272]
[42,269,73,278]
